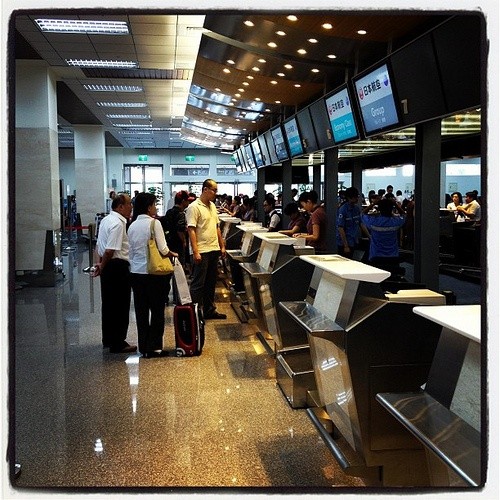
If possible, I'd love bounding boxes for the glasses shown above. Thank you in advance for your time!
[210,188,218,193]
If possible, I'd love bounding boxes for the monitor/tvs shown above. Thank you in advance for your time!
[232,12,483,174]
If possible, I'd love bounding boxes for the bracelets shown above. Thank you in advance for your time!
[300,232,301,235]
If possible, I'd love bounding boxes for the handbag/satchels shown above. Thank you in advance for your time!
[171,255,193,305]
[146,218,175,275]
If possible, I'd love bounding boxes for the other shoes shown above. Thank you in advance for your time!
[110,341,138,352]
[204,312,227,319]
[141,350,170,358]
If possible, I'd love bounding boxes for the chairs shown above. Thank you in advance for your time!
[438,200,480,269]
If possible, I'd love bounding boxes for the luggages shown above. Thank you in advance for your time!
[173,257,205,357]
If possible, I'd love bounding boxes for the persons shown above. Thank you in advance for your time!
[336,185,481,279]
[91,178,330,358]
